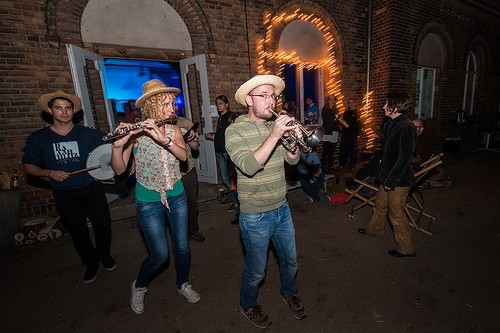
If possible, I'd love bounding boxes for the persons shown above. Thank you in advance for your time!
[338,97,360,169]
[282,94,318,125]
[321,94,341,174]
[170,102,206,243]
[226,112,241,225]
[223,73,308,329]
[21,89,118,282]
[357,91,419,257]
[204,94,237,212]
[110,78,203,315]
[112,99,143,128]
[406,118,435,206]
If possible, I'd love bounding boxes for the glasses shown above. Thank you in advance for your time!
[328,98,335,100]
[248,93,277,99]
[415,125,424,129]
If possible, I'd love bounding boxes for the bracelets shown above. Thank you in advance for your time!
[162,137,171,144]
[162,140,175,149]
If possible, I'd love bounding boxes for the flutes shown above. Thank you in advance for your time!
[102,115,177,141]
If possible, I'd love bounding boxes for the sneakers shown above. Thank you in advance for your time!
[175,281,200,304]
[239,304,271,328]
[82,255,118,285]
[131,279,149,314]
[281,291,307,319]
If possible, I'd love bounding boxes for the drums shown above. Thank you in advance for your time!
[87,143,136,192]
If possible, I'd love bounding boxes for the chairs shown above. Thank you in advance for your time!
[344,153,448,236]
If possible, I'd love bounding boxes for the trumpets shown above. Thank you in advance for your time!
[265,107,314,154]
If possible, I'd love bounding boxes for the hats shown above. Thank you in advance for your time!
[37,90,82,115]
[134,79,181,107]
[235,75,285,107]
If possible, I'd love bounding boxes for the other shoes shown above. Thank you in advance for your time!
[191,232,205,243]
[220,198,238,213]
[231,213,240,224]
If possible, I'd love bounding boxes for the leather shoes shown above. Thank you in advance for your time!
[358,228,366,234]
[388,250,415,257]
